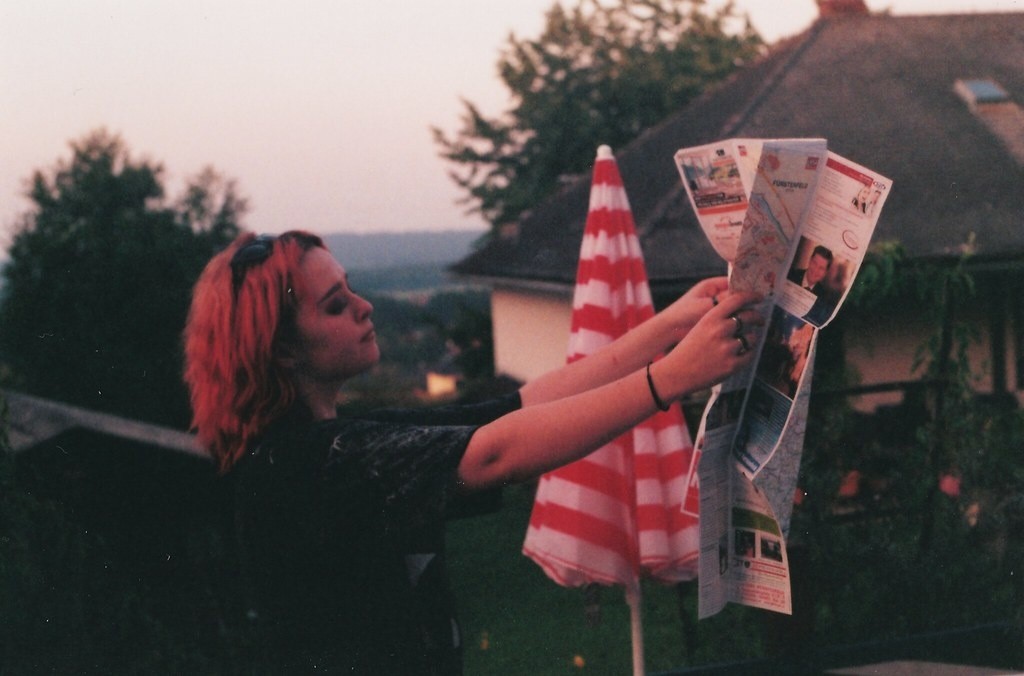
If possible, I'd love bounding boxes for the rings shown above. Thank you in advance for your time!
[712,295,718,306]
[734,335,748,355]
[729,312,742,332]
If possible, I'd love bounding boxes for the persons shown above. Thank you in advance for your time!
[179,230,767,676]
[714,235,853,574]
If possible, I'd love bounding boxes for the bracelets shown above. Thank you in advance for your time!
[646,362,669,412]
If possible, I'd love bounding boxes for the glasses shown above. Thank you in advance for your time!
[227,232,279,304]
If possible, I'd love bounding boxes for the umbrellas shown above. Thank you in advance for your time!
[519,142,709,676]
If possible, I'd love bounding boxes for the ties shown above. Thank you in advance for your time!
[804,286,811,291]
